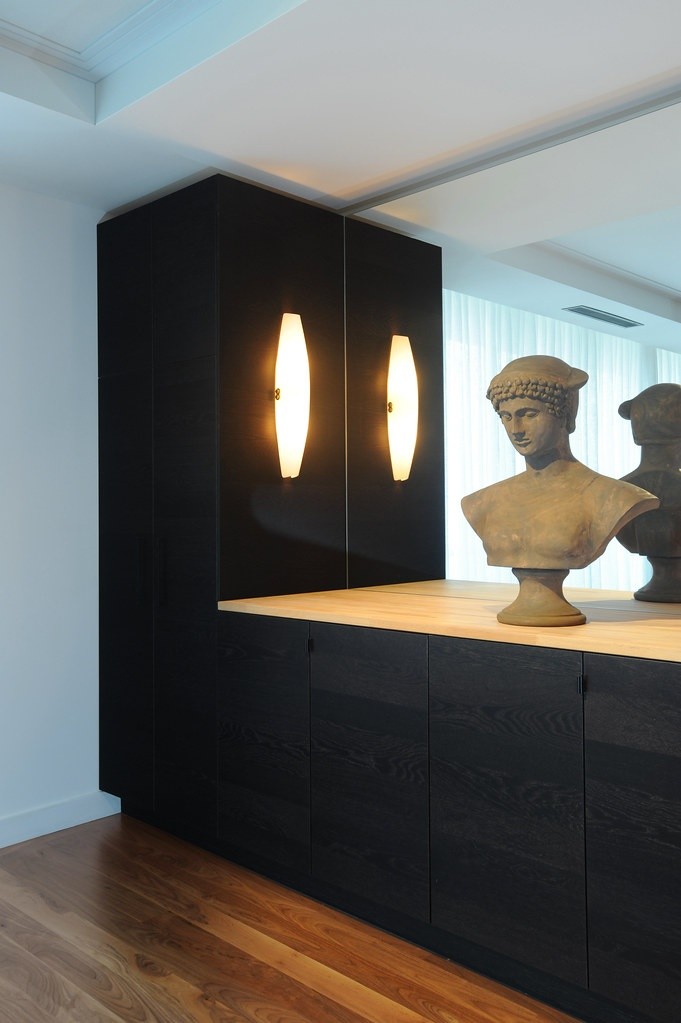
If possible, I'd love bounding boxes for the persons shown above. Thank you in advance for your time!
[615,383,681,558]
[460,355,659,570]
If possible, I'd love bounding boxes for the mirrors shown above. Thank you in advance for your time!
[345,103,681,589]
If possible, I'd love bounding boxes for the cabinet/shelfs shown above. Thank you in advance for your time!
[96,172,681,1023]
[345,215,447,591]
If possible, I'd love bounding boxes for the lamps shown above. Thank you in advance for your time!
[272,313,313,479]
[387,334,418,480]
